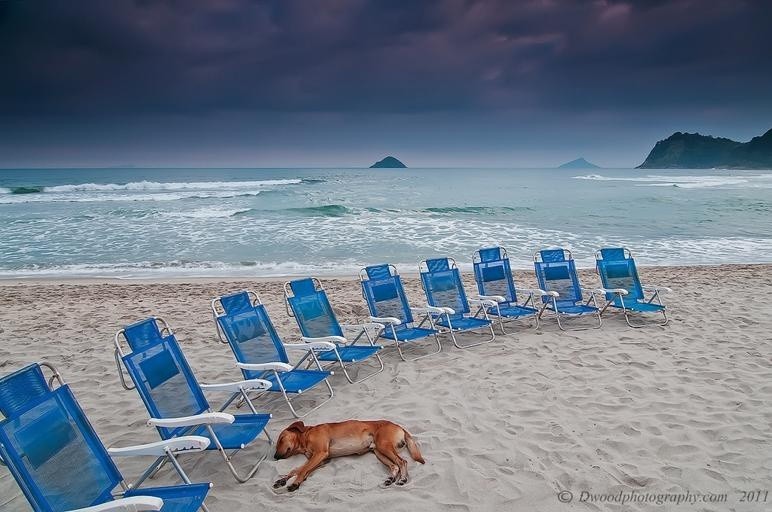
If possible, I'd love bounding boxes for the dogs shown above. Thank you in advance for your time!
[270,419,426,493]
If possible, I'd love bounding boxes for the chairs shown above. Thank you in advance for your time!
[111,316,275,484]
[358,263,442,363]
[592,246,669,331]
[210,291,337,415]
[418,256,497,347]
[532,249,603,333]
[283,275,385,386]
[0,360,215,512]
[470,246,541,337]
